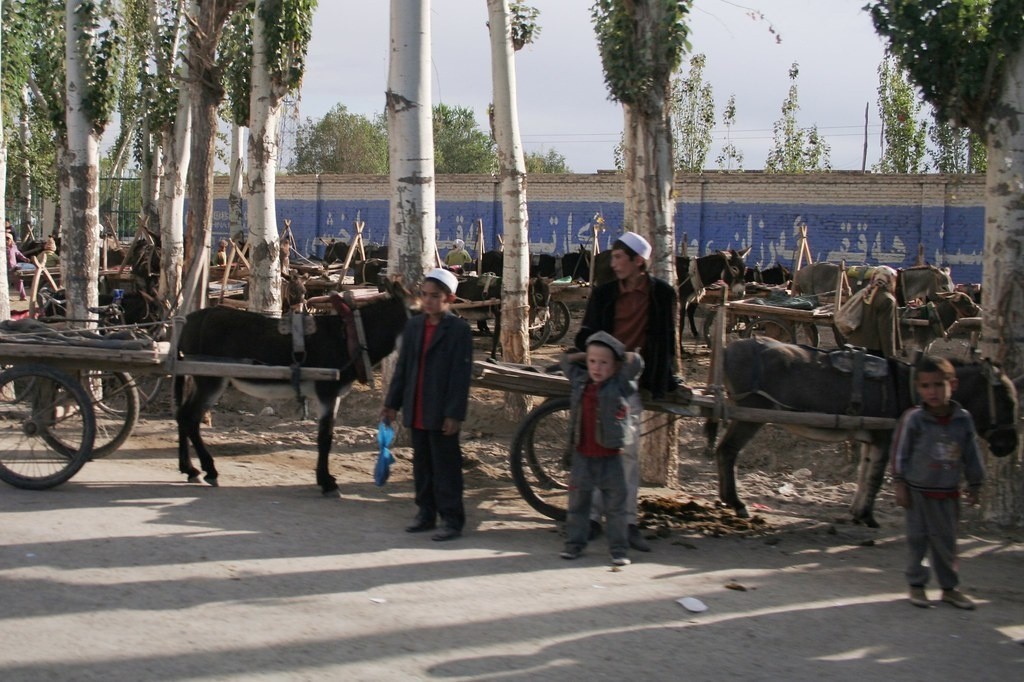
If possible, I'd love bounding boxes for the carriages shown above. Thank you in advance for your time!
[0,196,986,395]
[0,271,413,497]
[461,324,1024,531]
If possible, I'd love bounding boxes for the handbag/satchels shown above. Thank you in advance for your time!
[834,291,864,337]
[373,419,395,486]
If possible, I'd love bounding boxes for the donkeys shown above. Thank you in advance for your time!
[13,225,1019,530]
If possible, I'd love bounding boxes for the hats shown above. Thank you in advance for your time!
[426,268,459,294]
[619,232,652,259]
[585,331,626,356]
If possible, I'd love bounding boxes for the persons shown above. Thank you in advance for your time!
[380,267,473,540]
[895,356,986,609]
[559,331,646,565]
[573,232,693,551]
[444,239,473,267]
[36,237,61,290]
[5,217,32,302]
[835,264,906,360]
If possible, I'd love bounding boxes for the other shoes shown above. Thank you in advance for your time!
[558,543,582,558]
[909,587,930,608]
[404,516,436,532]
[589,522,601,540]
[630,531,652,551]
[942,590,976,609]
[431,526,462,540]
[609,551,631,565]
